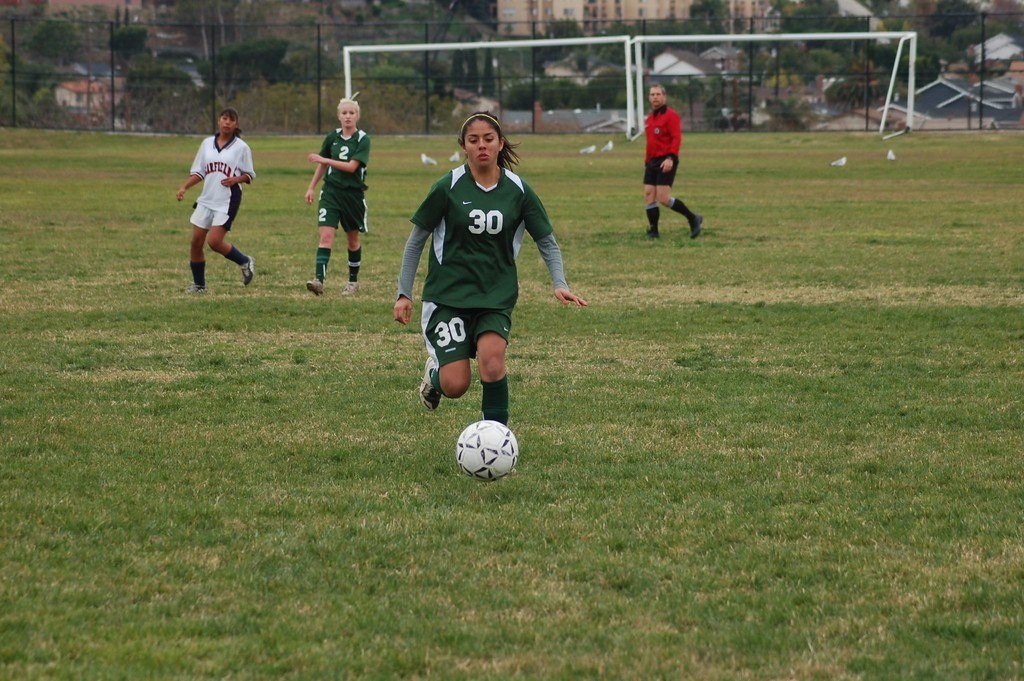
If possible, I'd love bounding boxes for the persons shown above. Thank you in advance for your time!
[643,83,704,241]
[392,113,588,422]
[306,98,372,295]
[176,108,256,294]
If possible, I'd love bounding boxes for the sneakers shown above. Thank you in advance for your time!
[341,281,359,295]
[645,226,660,237]
[419,357,441,411]
[184,283,208,292]
[306,279,324,295]
[240,256,254,286]
[689,214,704,238]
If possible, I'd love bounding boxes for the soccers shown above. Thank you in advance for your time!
[456,419,521,482]
